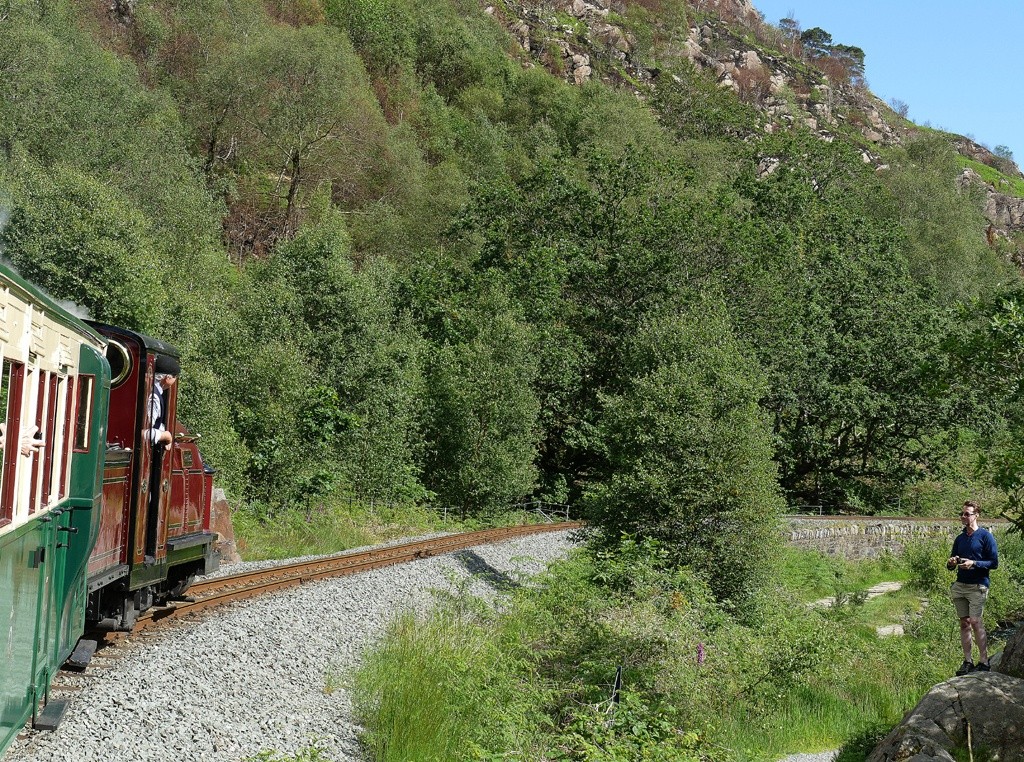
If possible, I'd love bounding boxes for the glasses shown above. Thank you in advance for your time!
[959,512,976,516]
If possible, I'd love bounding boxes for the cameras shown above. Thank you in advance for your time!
[956,558,962,564]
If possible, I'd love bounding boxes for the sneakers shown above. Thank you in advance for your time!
[971,658,991,671]
[956,659,974,676]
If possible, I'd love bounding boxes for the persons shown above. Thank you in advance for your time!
[145,355,180,564]
[0,422,7,450]
[21,426,45,457]
[947,501,998,675]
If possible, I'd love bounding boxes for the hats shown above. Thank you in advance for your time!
[155,356,180,374]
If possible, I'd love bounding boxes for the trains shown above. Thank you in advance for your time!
[0,261,224,762]
